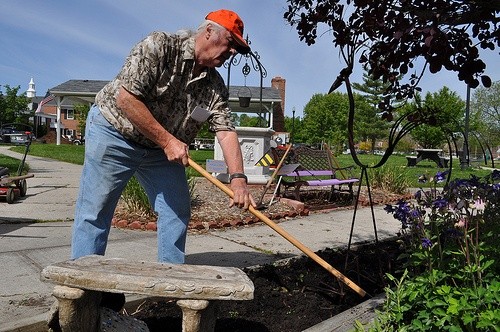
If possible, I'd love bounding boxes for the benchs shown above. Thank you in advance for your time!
[271,144,361,204]
[40,254,255,332]
[439,157,451,168]
[406,156,418,167]
[469,154,483,169]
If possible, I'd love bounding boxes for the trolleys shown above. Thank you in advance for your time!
[0,123,35,204]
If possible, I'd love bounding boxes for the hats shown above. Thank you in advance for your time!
[204,9,251,54]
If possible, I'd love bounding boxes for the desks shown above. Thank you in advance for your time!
[416,148,442,168]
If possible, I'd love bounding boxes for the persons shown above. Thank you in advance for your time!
[69,10,258,265]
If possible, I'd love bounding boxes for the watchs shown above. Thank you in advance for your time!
[227,174,247,184]
